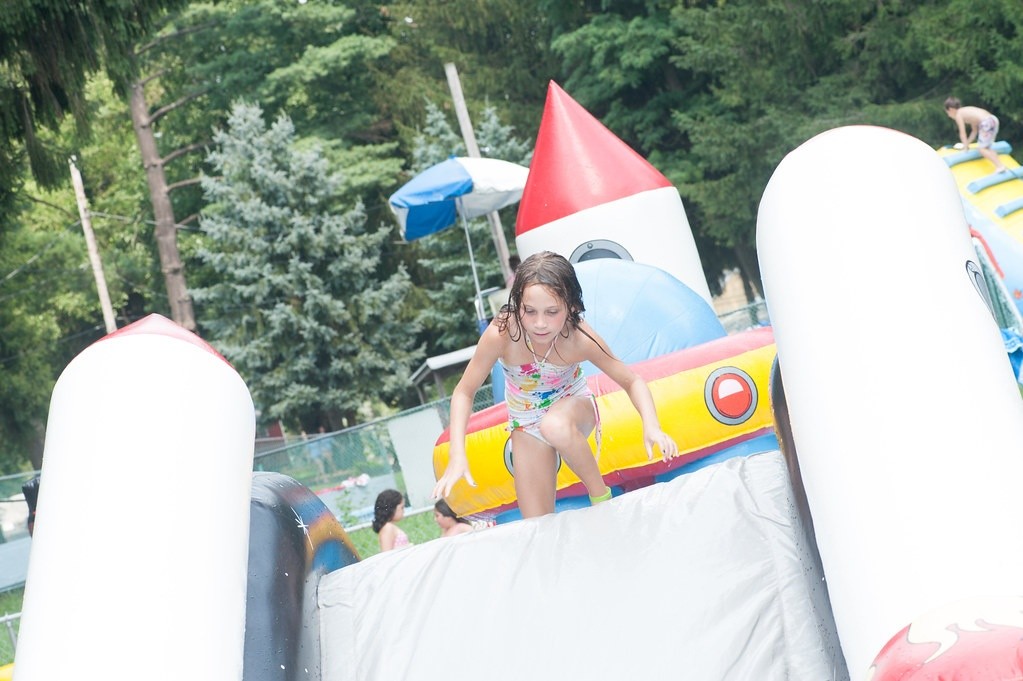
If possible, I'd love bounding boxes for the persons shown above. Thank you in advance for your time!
[373,489,411,552]
[432,250,680,519]
[943,97,1006,175]
[433,499,474,538]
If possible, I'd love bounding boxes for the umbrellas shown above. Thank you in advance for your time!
[387,155,529,320]
